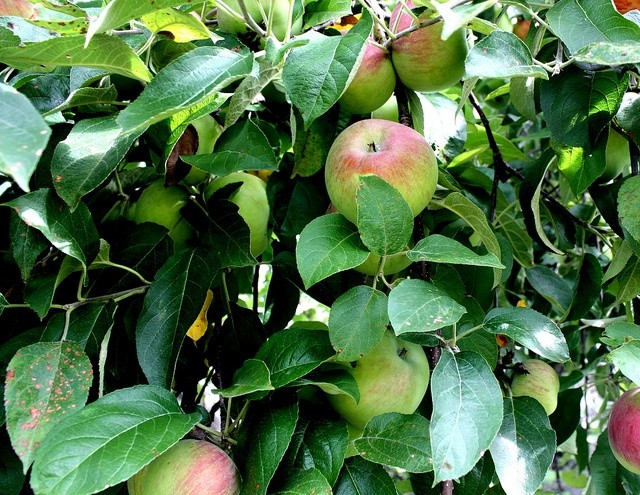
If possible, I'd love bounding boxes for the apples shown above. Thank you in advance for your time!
[511,359,560,417]
[325,201,416,276]
[388,0,466,92]
[329,330,431,431]
[509,78,544,123]
[337,30,397,115]
[205,172,272,258]
[613,0,640,15]
[177,114,222,186]
[595,130,630,184]
[512,16,531,41]
[325,118,438,228]
[132,180,194,244]
[607,387,640,474]
[217,0,303,50]
[126,439,242,494]
